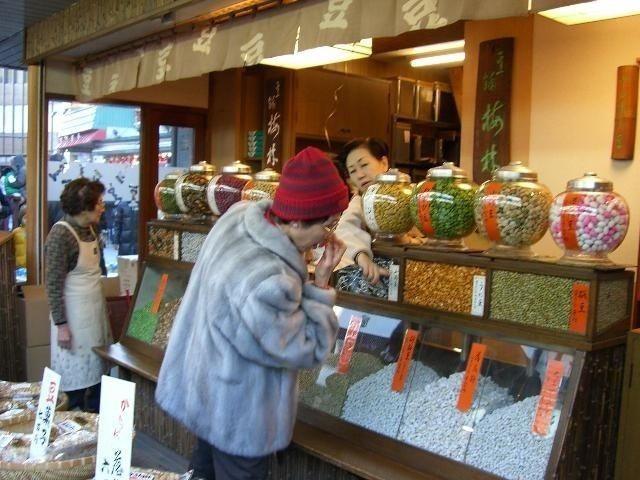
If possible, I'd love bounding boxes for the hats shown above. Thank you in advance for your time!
[270,146,350,222]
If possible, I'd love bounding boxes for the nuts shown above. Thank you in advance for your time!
[546,191,629,256]
[153,171,279,219]
[402,258,485,317]
[361,178,478,241]
[489,267,629,332]
[473,180,553,248]
[147,225,208,265]
[333,256,396,300]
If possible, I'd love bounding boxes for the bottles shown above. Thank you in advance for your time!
[548,172,631,265]
[361,166,420,248]
[409,161,482,252]
[173,160,215,225]
[206,160,251,226]
[473,159,554,258]
[154,167,183,222]
[241,167,281,202]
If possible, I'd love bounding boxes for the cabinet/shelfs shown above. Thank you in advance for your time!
[88,222,632,480]
[281,65,391,172]
[382,75,462,181]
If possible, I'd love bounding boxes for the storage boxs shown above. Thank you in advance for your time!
[101,276,122,298]
[15,347,49,384]
[13,284,52,347]
[117,255,140,296]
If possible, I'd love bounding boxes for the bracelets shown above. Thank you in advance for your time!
[350,251,370,264]
[53,321,68,325]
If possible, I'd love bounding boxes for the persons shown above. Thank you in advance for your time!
[42,176,117,414]
[153,146,350,480]
[334,135,422,288]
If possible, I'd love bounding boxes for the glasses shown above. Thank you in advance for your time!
[321,213,342,234]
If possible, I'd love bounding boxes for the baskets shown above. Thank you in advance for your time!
[0,410,137,480]
[0,383,69,412]
